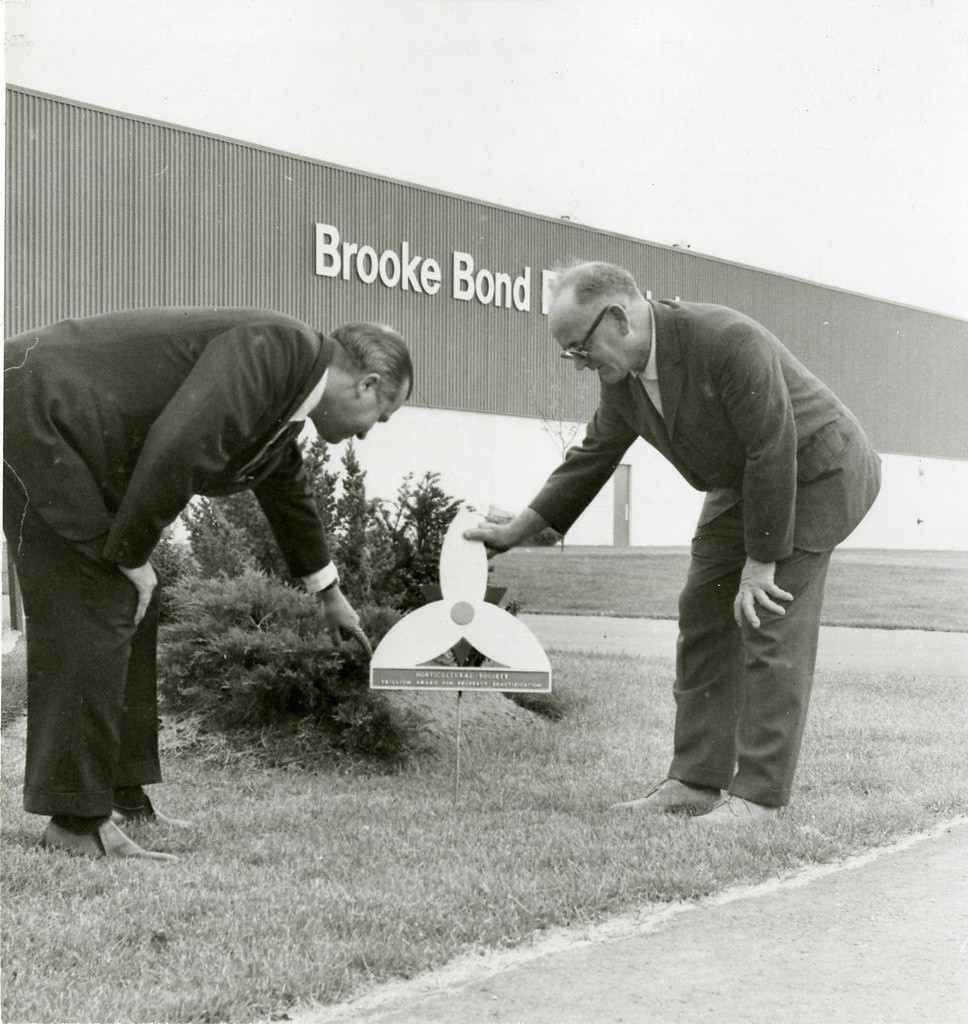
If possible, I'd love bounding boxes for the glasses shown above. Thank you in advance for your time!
[560,304,627,360]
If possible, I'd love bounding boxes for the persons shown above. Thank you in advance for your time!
[460,259,880,823]
[3,307,413,860]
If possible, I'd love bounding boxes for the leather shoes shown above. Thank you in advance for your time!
[109,795,197,837]
[688,795,784,825]
[609,780,723,817]
[35,819,177,867]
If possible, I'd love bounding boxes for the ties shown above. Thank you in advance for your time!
[232,419,307,481]
